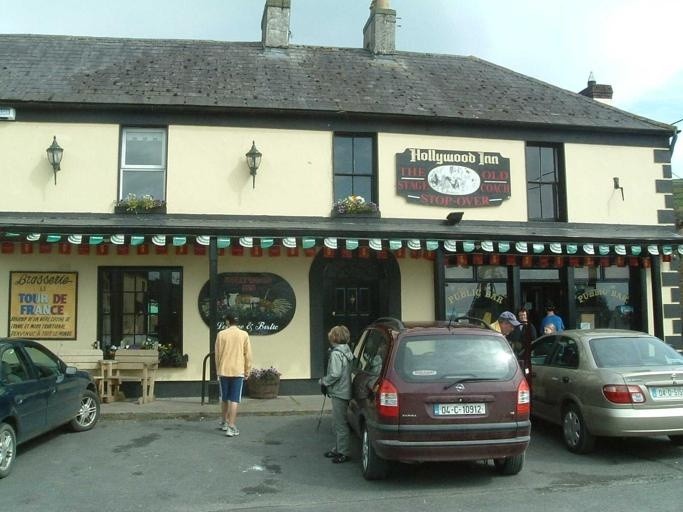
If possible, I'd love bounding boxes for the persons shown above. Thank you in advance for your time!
[488,311,532,388]
[215,314,253,437]
[512,308,537,341]
[543,323,556,334]
[318,325,354,463]
[539,302,565,335]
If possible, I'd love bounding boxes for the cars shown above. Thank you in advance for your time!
[518,328,681,450]
[0,336,99,480]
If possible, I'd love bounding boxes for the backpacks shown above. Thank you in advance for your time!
[329,349,369,400]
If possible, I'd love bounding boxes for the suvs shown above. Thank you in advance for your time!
[353,314,532,481]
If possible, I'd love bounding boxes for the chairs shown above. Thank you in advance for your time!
[103,349,160,405]
[58,349,106,401]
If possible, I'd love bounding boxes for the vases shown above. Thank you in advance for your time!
[245,381,280,399]
[115,202,166,214]
[331,208,381,218]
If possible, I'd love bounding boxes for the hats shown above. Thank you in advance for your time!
[222,311,239,320]
[500,311,523,327]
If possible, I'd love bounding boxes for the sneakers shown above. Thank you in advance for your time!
[226,426,239,436]
[218,422,228,430]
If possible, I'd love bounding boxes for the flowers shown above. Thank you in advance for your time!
[244,366,281,383]
[333,194,377,214]
[115,193,163,210]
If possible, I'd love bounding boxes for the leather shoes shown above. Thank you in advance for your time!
[324,451,338,457]
[332,456,350,463]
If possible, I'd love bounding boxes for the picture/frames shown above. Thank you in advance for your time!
[8,271,78,339]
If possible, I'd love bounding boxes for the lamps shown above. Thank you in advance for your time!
[246,140,262,189]
[47,136,63,184]
